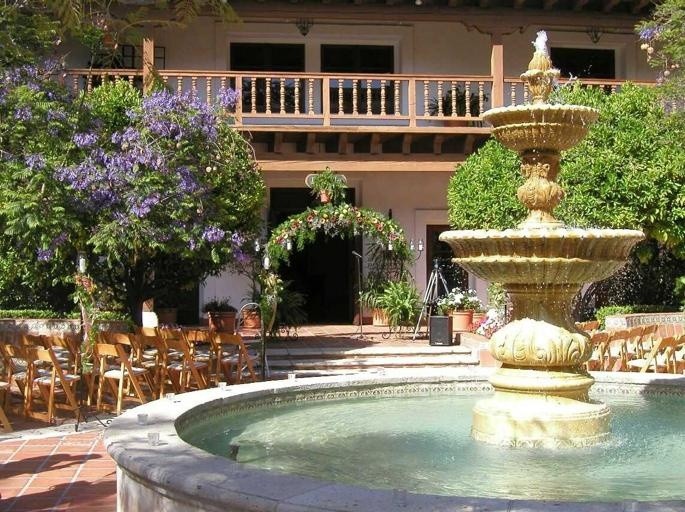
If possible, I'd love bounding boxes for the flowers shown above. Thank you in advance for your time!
[263,201,409,268]
[437,287,482,312]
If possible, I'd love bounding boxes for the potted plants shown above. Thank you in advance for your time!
[236,261,261,328]
[309,166,348,203]
[201,296,238,333]
[253,279,309,335]
[377,277,423,325]
[355,274,393,326]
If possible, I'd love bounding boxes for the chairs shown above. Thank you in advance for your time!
[0,326,260,432]
[574,320,685,374]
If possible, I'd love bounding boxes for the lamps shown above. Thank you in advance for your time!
[409,237,424,262]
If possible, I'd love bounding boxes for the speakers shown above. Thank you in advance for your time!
[428,316,453,346]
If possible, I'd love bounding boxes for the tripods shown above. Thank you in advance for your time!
[412,258,449,341]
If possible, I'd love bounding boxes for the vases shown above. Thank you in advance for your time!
[448,309,474,331]
[156,307,178,324]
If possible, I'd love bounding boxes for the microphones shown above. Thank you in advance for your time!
[351,250,362,258]
